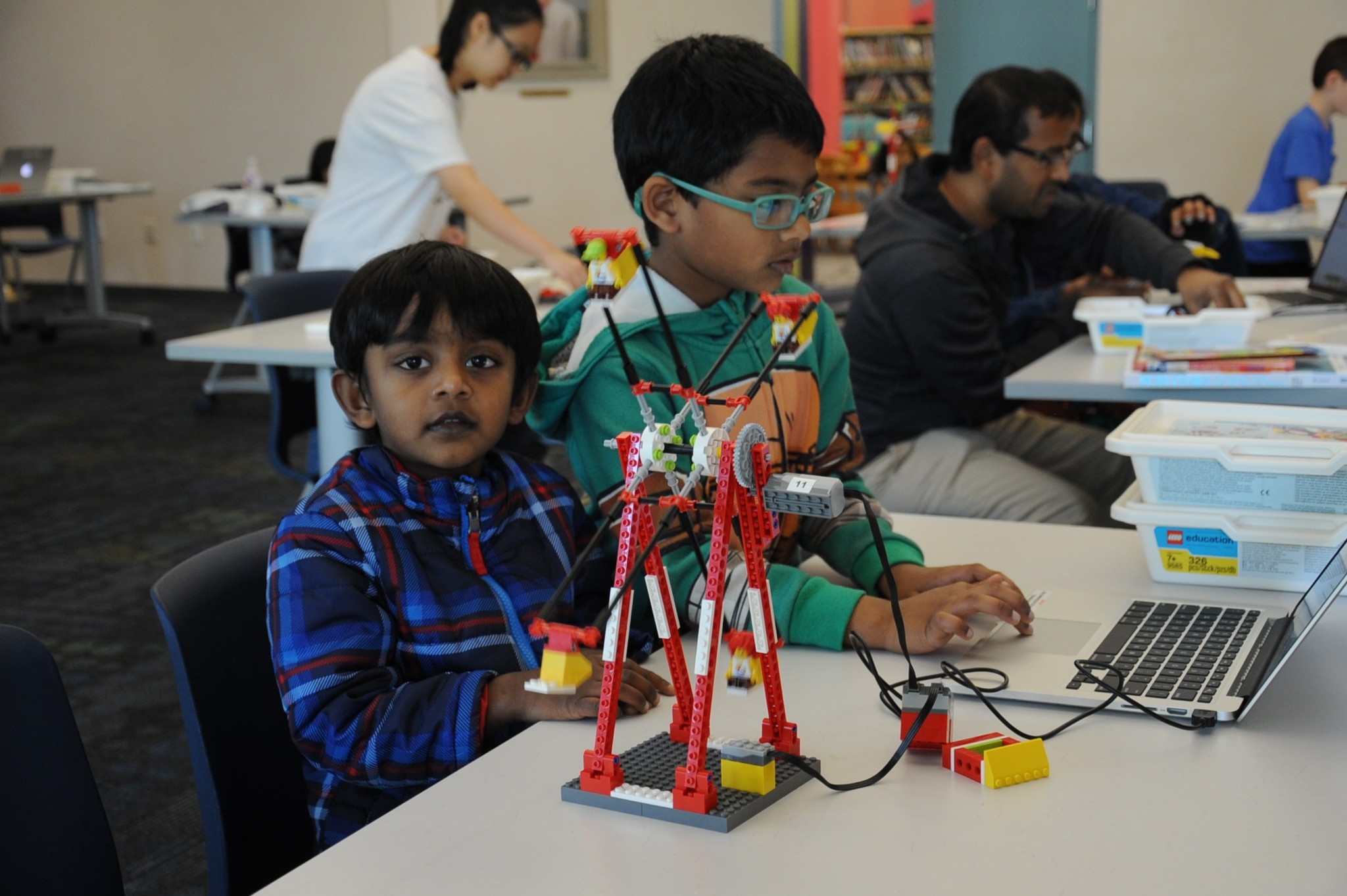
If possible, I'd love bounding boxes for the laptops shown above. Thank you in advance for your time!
[945,540,1347,720]
[1243,193,1347,313]
[0,147,55,193]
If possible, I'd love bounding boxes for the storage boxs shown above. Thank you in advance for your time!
[1104,398,1347,593]
[1071,293,1270,351]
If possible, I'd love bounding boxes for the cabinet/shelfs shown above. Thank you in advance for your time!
[834,30,941,185]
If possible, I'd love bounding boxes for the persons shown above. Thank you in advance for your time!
[292,0,590,296]
[501,33,1034,654]
[1239,34,1347,276]
[840,68,1248,524]
[261,242,681,857]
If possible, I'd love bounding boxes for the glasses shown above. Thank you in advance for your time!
[1014,131,1089,169]
[633,172,835,231]
[498,29,533,72]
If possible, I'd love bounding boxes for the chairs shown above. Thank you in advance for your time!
[0,623,122,896]
[0,204,82,315]
[250,267,353,482]
[151,526,321,896]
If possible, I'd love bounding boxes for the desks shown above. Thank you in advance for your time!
[1004,276,1347,404]
[252,511,1347,894]
[1240,201,1338,240]
[0,184,153,338]
[184,191,322,409]
[165,267,573,482]
